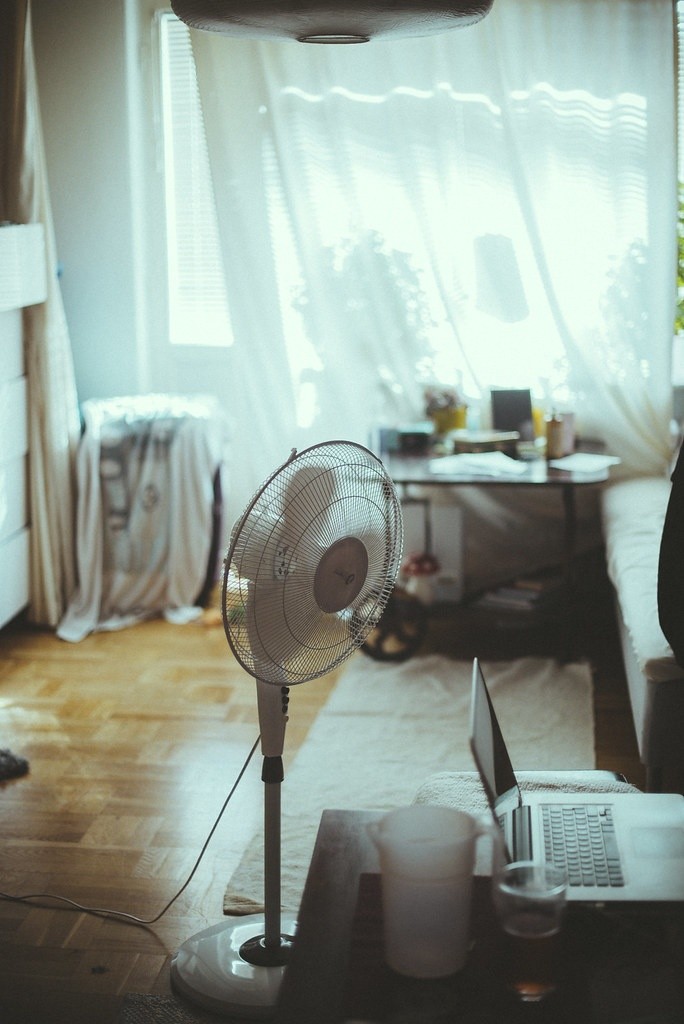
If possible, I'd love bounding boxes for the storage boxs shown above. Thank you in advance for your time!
[453,433,520,460]
[379,426,431,457]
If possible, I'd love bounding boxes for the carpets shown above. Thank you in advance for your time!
[222,654,597,910]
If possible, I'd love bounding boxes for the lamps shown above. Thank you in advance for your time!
[171,0,494,44]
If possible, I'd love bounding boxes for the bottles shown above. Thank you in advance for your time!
[559,412,575,455]
[546,408,565,459]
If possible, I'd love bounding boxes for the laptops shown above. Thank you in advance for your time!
[468,658,684,908]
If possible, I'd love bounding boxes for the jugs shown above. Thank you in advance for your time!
[365,805,506,978]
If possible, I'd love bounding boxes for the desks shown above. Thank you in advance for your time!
[342,454,622,663]
[270,810,684,1024]
[0,222,50,631]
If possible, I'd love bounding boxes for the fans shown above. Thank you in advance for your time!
[168,440,406,1024]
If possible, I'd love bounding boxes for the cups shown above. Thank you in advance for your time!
[493,862,569,1003]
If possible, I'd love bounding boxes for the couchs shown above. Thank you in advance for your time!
[598,477,684,796]
